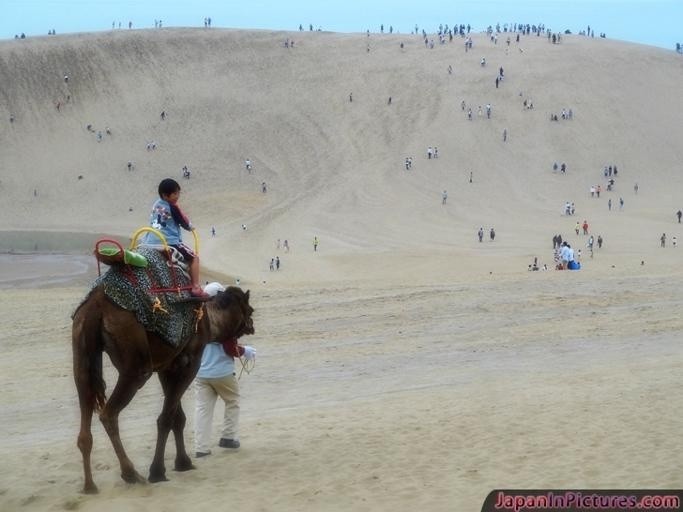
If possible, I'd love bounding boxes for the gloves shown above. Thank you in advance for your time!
[243,346,256,359]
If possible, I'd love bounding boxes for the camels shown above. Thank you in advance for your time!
[72,267,256,494]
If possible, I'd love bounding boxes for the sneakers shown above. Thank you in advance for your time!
[219,438,240,448]
[196,450,211,458]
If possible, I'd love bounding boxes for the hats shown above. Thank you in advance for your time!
[204,282,226,297]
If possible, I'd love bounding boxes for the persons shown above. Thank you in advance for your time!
[276,256,279,269]
[660,233,666,246]
[283,239,289,253]
[672,236,678,247]
[365,22,626,274]
[109,14,322,48]
[633,181,639,194]
[140,176,209,297]
[269,257,275,270]
[675,43,680,55]
[193,283,256,460]
[313,236,319,251]
[244,157,250,170]
[241,223,247,231]
[210,225,215,237]
[52,73,192,180]
[677,209,681,222]
[261,182,268,193]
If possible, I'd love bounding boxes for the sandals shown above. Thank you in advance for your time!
[191,288,209,297]
